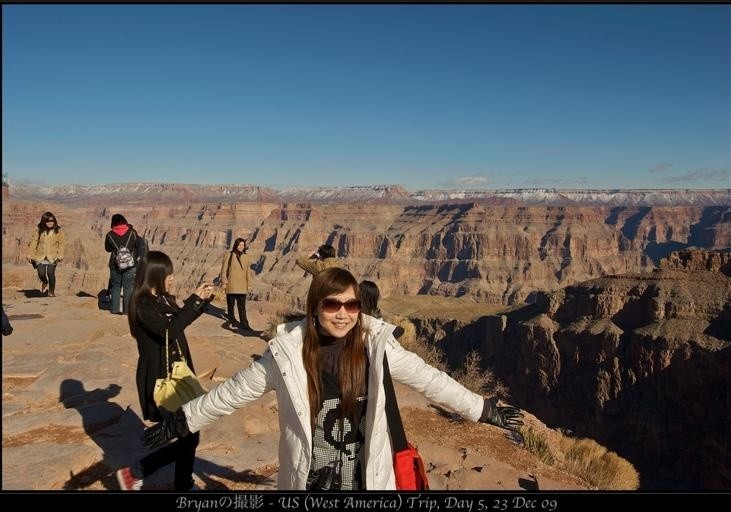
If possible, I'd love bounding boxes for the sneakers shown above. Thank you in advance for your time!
[116,465,143,490]
[49,293,56,297]
[40,280,48,294]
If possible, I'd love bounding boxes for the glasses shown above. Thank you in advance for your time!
[46,220,53,223]
[316,299,364,314]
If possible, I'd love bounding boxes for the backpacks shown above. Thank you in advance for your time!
[107,230,135,271]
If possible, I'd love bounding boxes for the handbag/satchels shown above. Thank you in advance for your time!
[212,273,229,287]
[31,259,38,269]
[392,442,429,491]
[153,360,207,417]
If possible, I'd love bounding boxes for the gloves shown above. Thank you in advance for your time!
[478,397,524,431]
[139,407,189,450]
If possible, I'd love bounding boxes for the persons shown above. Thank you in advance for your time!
[26,211,66,297]
[104,213,138,315]
[1,309,13,336]
[115,250,216,491]
[358,281,386,321]
[139,267,525,491]
[295,244,346,279]
[219,238,255,331]
[129,223,149,256]
